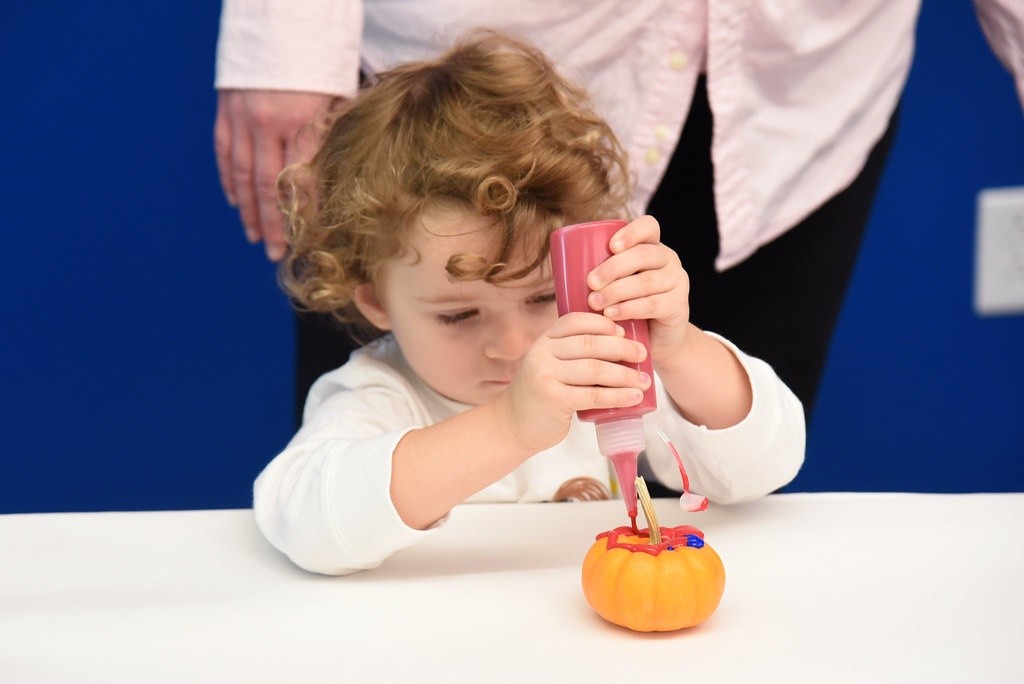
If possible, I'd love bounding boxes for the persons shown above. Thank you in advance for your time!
[251,27,803,578]
[214,0,1024,499]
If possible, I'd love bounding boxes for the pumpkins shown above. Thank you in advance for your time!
[582,476,725,632]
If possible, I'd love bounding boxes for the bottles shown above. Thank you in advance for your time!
[550,219,657,519]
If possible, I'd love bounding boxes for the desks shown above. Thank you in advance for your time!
[0,491,1024,684]
